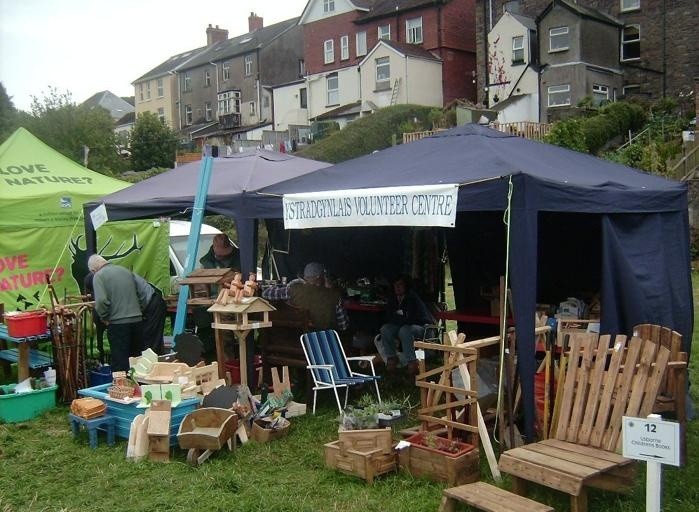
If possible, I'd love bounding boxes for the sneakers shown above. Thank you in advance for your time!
[407,360,418,378]
[387,356,400,375]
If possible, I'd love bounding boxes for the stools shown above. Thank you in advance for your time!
[69,413,117,448]
[439,481,554,511]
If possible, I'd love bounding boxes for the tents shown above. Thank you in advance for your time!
[0,123,172,360]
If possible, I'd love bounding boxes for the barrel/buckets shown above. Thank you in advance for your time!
[224,355,262,387]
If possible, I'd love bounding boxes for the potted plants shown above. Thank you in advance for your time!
[338,392,418,455]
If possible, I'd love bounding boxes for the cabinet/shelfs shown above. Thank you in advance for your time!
[0,303,54,384]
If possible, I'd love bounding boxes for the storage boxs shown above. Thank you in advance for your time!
[2,309,48,338]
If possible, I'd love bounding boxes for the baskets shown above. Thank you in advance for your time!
[107,385,135,400]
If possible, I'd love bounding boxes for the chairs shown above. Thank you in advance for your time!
[495,325,688,511]
[260,298,383,418]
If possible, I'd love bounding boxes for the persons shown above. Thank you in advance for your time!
[85,271,168,357]
[380,272,435,377]
[261,261,352,343]
[199,232,241,297]
[87,254,145,374]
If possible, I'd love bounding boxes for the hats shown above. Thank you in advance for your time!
[213,234,233,256]
